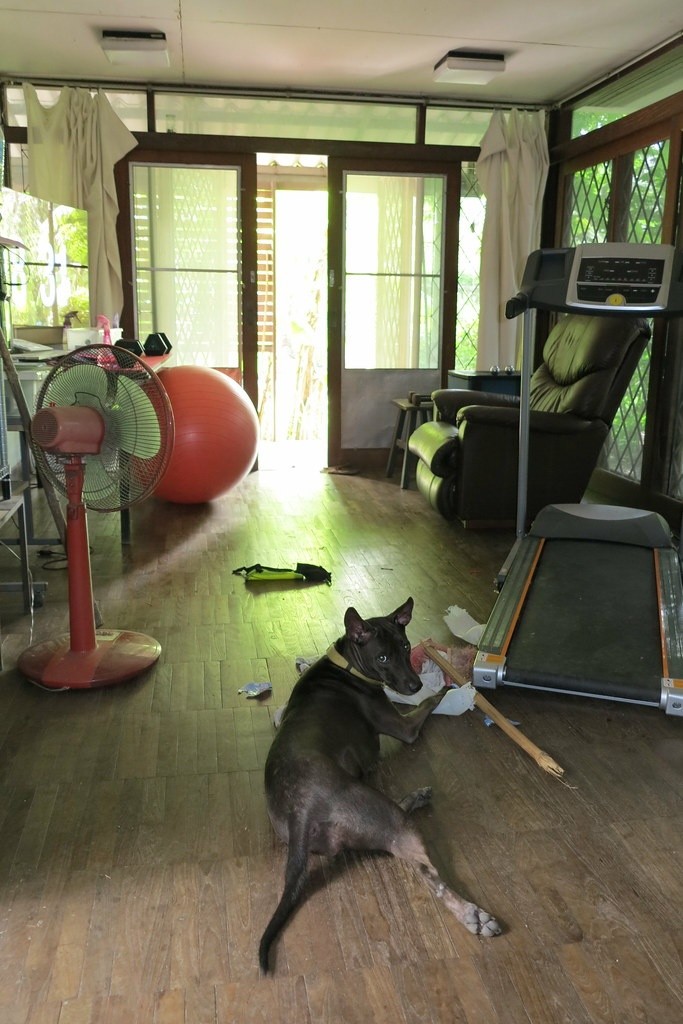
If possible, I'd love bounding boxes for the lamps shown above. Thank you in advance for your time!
[100,30,170,68]
[432,49,506,86]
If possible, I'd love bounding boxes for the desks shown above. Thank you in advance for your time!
[1,348,175,544]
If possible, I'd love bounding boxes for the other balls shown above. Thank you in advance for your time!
[131,365,260,504]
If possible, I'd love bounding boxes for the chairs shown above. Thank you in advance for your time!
[408,313,651,530]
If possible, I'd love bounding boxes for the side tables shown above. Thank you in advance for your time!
[447,369,522,397]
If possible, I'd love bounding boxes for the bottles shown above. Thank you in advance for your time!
[60,311,82,350]
[95,314,116,367]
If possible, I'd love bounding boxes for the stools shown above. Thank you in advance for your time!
[386,399,433,488]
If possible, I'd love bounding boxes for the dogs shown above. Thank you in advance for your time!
[258,596,502,977]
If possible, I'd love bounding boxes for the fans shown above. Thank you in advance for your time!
[17,344,176,691]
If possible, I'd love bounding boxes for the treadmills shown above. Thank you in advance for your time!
[473,242,683,716]
